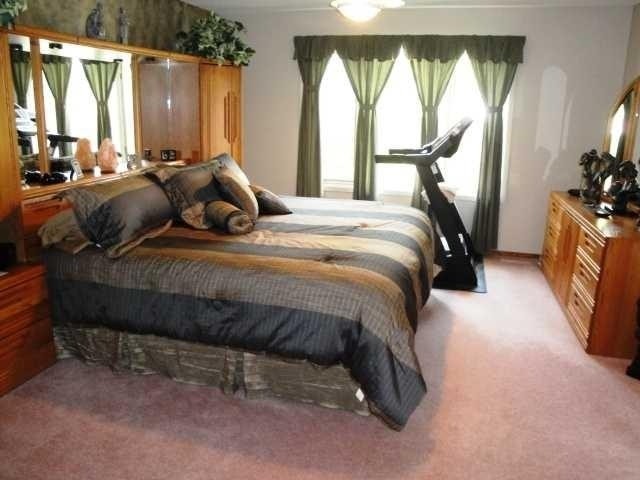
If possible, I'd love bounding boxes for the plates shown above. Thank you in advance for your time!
[569,189,584,198]
[591,208,612,217]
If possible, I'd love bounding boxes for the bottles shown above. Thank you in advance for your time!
[93,163,101,177]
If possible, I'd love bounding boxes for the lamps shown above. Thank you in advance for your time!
[330,0,405,21]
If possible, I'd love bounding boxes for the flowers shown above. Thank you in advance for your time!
[176,11,255,66]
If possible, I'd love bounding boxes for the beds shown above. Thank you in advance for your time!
[44,194,435,416]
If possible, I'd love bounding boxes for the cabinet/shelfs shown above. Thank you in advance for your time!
[136,62,241,168]
[538,192,640,361]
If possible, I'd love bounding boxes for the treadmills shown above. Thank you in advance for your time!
[11,100,79,158]
[374,120,481,291]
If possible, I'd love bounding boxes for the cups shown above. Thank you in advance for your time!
[127,154,137,169]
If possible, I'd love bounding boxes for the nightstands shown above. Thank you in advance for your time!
[0,262,56,399]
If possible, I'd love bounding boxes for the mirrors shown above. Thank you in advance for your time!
[600,75,640,204]
[7,32,137,184]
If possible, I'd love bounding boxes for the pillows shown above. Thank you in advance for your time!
[211,162,259,223]
[143,152,250,230]
[247,183,293,215]
[52,175,175,261]
